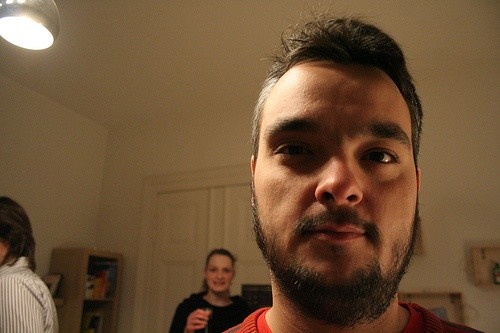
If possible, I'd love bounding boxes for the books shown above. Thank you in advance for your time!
[86,265,117,299]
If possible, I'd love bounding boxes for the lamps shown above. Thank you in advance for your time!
[0,0,60,51]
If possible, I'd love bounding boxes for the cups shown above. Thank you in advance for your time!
[196,308,212,333]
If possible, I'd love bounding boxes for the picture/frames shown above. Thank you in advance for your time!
[398,292,465,325]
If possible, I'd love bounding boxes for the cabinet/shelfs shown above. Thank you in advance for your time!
[54,247,122,333]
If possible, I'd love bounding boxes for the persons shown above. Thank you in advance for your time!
[219,16,484,333]
[169,248,253,333]
[0,196,60,333]
